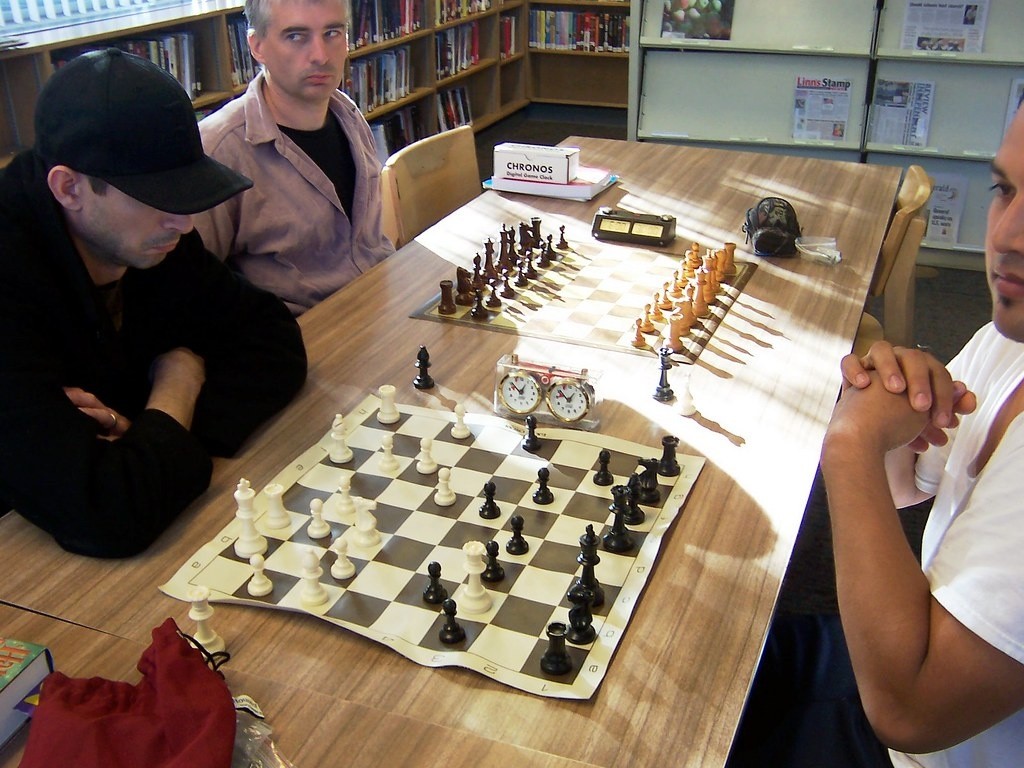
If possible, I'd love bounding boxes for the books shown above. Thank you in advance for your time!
[490,166,612,198]
[50,14,264,124]
[0,637,56,754]
[335,0,516,167]
[482,175,619,203]
[527,9,630,54]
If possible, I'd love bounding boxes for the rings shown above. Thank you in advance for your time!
[915,342,932,353]
[106,413,117,433]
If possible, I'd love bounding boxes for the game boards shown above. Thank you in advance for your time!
[159,391,706,698]
[407,230,760,365]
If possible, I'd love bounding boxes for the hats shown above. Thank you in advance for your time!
[32,45,255,217]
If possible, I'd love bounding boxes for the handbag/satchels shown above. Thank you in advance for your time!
[743,196,804,257]
[14,617,238,768]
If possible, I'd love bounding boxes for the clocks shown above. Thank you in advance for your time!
[545,380,591,424]
[498,373,542,414]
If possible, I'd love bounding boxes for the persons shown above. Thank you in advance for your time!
[965,5,977,25]
[918,38,963,52]
[0,48,308,559]
[724,91,1024,768]
[188,0,400,322]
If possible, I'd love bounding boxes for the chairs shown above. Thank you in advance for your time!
[377,125,481,255]
[849,162,937,360]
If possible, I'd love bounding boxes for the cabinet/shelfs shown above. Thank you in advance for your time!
[625,0,1023,275]
[0,1,632,173]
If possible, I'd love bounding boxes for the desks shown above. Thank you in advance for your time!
[0,135,904,768]
